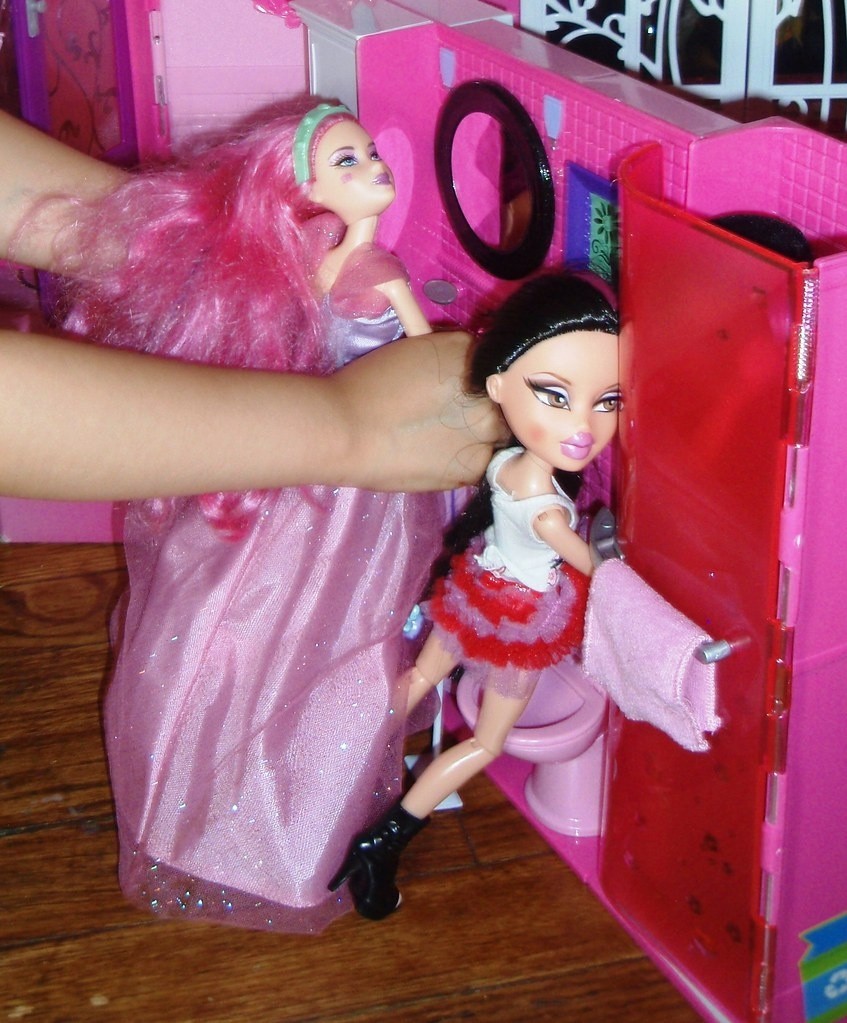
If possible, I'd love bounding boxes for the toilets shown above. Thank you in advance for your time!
[455,653,608,838]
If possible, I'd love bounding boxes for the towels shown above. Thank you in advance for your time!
[580,556,720,752]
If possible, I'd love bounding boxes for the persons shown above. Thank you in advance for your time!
[16,90,476,937]
[0,108,514,500]
[327,272,620,919]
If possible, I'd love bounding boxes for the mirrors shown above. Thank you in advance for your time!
[434,78,554,280]
